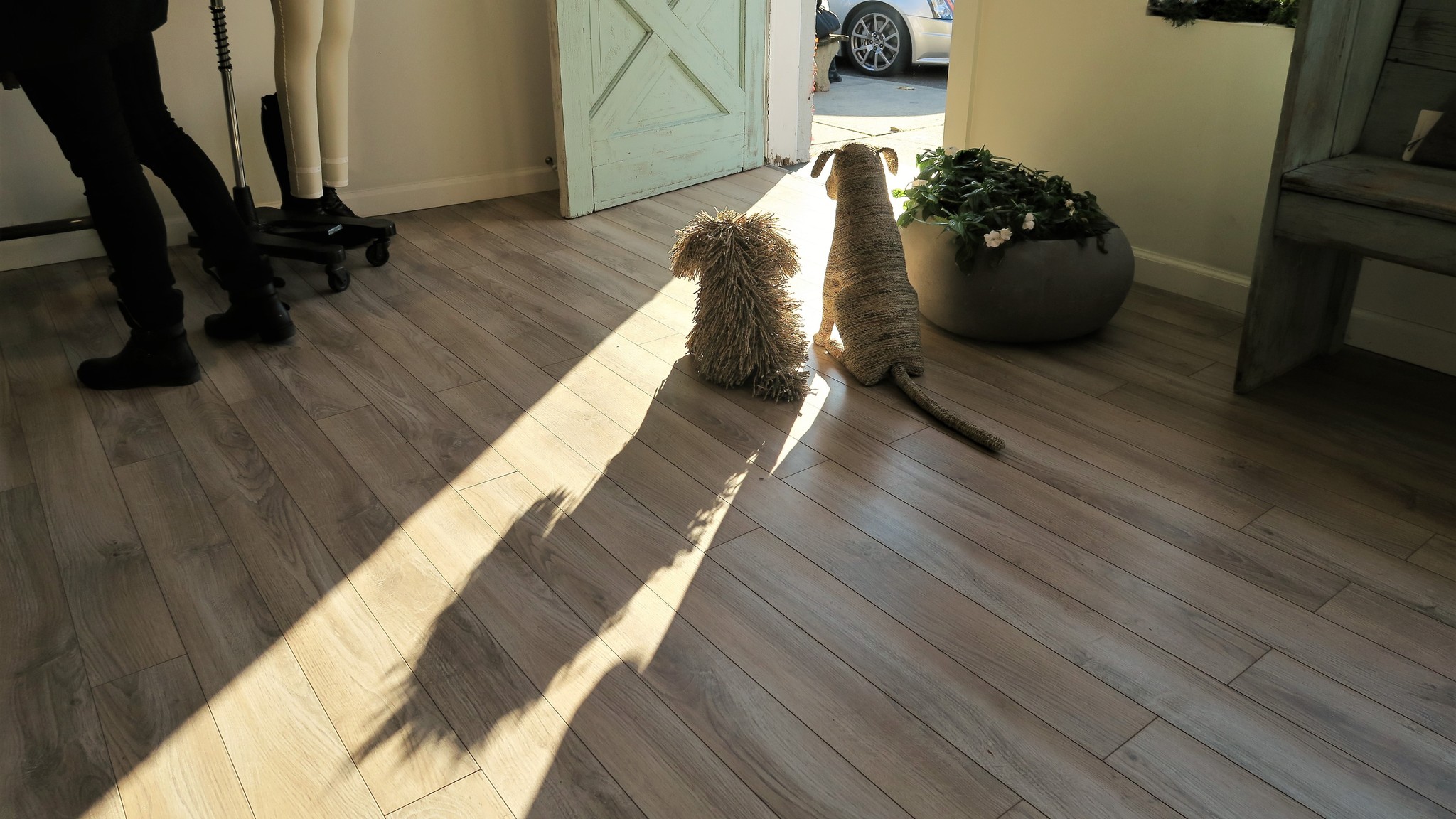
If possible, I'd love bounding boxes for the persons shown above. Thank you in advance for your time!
[272,1,361,222]
[815,0,842,84]
[0,1,294,393]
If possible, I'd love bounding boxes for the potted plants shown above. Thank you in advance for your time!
[894,144,1135,345]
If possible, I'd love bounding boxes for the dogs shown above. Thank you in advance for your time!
[810,141,1005,450]
[670,208,813,400]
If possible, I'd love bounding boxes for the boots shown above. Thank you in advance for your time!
[829,57,842,83]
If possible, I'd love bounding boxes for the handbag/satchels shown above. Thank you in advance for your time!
[816,6,841,39]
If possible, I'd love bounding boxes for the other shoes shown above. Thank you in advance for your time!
[282,189,370,245]
[76,322,200,391]
[205,281,297,344]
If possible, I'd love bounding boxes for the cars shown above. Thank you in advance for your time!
[819,0,955,76]
